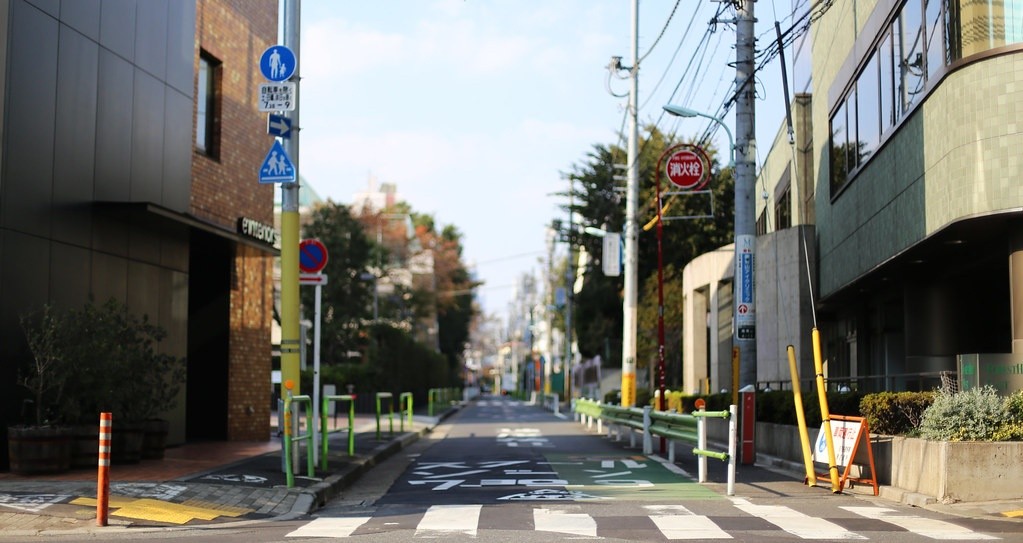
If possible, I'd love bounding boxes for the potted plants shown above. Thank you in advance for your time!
[8,292,190,476]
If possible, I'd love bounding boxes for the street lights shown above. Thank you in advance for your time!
[547,304,573,408]
[360,273,379,357]
[582,226,640,420]
[662,103,756,466]
